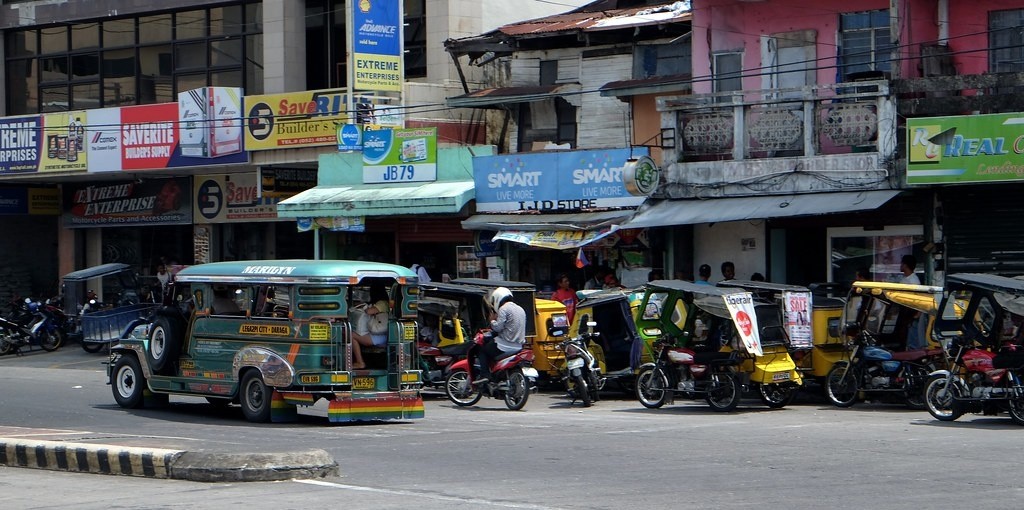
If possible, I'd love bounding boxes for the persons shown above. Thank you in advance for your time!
[895,254,920,290]
[551,275,578,326]
[350,285,390,370]
[155,264,172,291]
[720,261,738,282]
[584,267,623,289]
[855,266,875,323]
[475,287,527,380]
[693,264,715,286]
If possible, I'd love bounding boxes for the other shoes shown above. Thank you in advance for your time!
[352,362,365,370]
[472,375,489,385]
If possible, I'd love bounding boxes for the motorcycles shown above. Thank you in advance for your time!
[1,262,1024,427]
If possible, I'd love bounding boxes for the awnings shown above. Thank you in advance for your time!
[618,188,903,227]
[460,211,636,232]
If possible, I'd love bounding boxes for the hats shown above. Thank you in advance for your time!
[605,274,613,284]
[699,264,711,272]
[555,272,568,280]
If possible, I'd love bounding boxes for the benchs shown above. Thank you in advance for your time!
[351,344,396,369]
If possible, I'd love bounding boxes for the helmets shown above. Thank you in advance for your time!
[490,287,513,314]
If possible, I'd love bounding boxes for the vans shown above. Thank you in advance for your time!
[99,258,427,425]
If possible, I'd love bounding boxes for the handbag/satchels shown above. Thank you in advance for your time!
[367,312,389,335]
[349,303,371,337]
